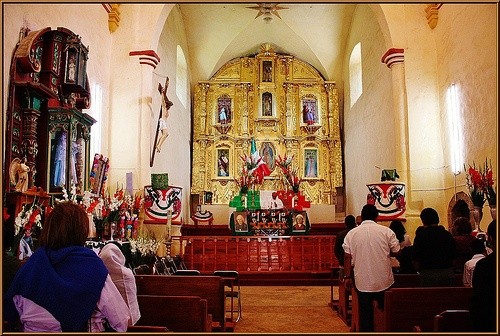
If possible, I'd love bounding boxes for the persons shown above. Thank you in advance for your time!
[6,203,141,333]
[341,204,400,332]
[293,214,306,230]
[236,215,247,231]
[388,220,411,249]
[335,215,357,266]
[411,206,497,288]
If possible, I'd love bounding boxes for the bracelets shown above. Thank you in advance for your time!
[343,275,352,279]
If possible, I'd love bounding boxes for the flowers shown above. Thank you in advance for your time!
[276,153,294,164]
[234,177,252,187]
[15,183,161,256]
[240,153,262,164]
[289,177,302,185]
[464,157,496,205]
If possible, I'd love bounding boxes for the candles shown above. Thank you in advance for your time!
[167,209,171,236]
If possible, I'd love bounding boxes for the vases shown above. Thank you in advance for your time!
[293,186,298,192]
[489,207,497,219]
[242,185,248,191]
[247,164,252,170]
[282,165,287,168]
[472,208,484,233]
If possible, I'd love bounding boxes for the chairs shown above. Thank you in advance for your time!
[177,269,242,323]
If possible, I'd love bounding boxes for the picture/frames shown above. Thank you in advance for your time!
[216,147,230,177]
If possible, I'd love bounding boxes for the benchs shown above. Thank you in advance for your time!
[329,267,473,332]
[99,275,223,333]
